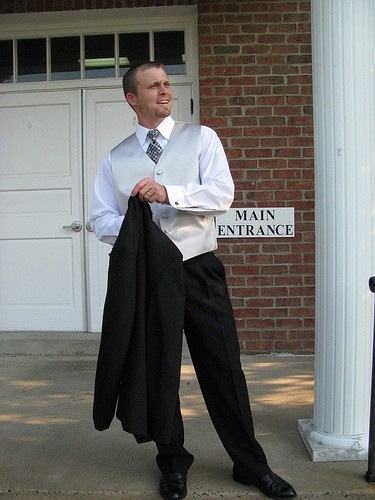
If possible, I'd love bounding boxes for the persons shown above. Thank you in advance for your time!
[88,61,300,500]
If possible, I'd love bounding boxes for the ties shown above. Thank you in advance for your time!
[146,129,164,165]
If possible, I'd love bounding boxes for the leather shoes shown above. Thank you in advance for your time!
[159,470,187,500]
[232,468,297,500]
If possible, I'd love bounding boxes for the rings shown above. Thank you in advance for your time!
[147,192,151,197]
